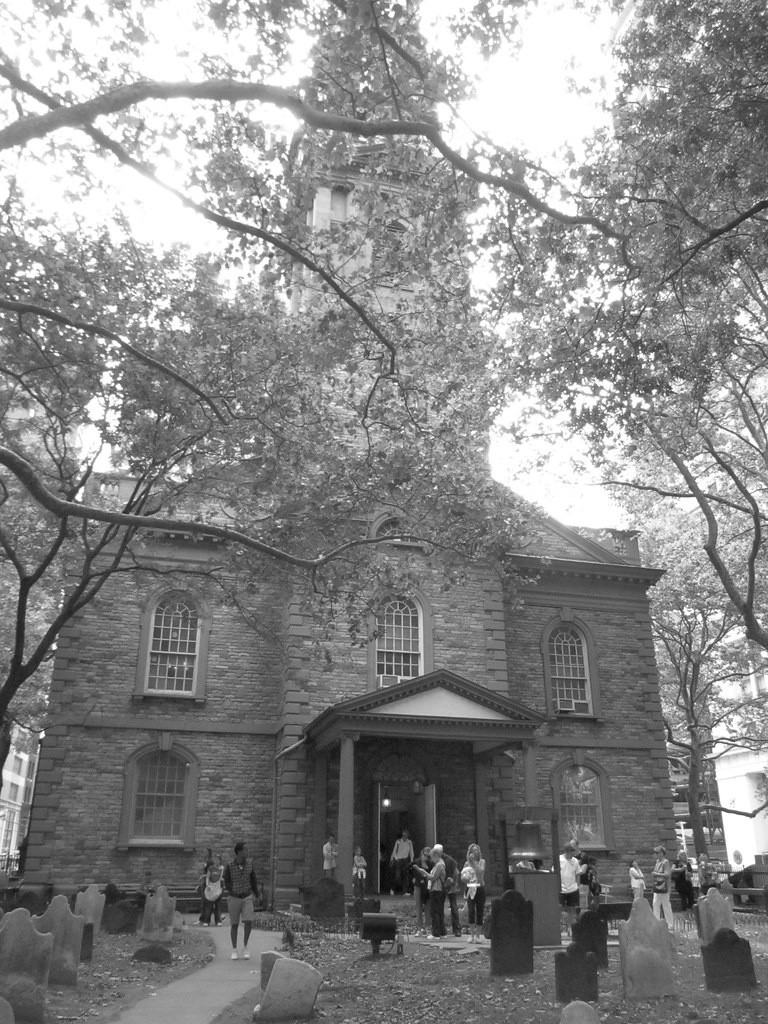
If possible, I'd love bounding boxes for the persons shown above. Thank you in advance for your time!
[413,844,461,940]
[464,844,486,943]
[322,835,337,878]
[193,848,226,927]
[698,853,717,895]
[352,847,368,901]
[552,839,600,930]
[516,859,536,871]
[389,830,414,895]
[652,846,674,933]
[223,842,262,960]
[629,859,646,898]
[672,850,693,911]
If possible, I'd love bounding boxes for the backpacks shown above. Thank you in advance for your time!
[442,877,454,895]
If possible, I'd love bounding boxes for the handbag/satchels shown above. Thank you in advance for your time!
[204,881,223,902]
[671,870,685,880]
[389,855,397,868]
[652,863,668,894]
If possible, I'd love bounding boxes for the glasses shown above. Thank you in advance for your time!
[654,852,660,854]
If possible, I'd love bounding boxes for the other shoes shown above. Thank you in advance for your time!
[427,934,441,940]
[390,888,394,896]
[194,921,201,926]
[467,937,481,944]
[216,922,222,926]
[203,923,208,927]
[220,914,226,920]
[441,934,447,939]
[231,949,238,960]
[402,892,411,897]
[415,931,421,937]
[455,931,461,937]
[242,949,250,959]
